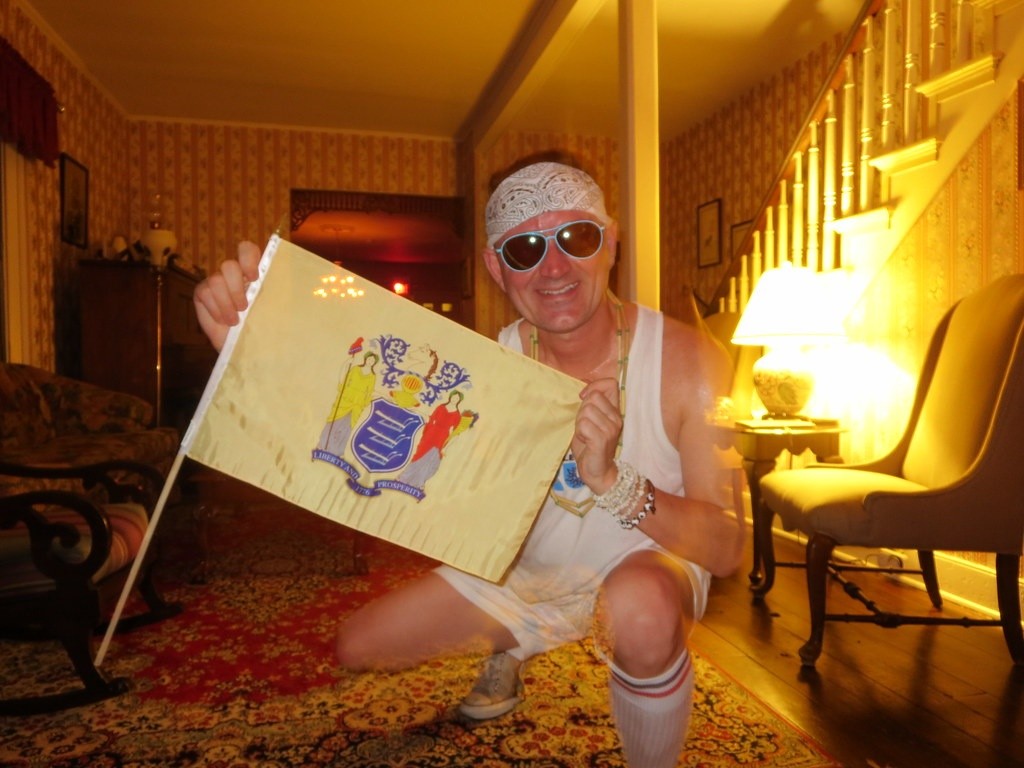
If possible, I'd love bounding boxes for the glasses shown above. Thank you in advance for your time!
[494,219,605,273]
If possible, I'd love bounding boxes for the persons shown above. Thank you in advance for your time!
[194,151,756,767]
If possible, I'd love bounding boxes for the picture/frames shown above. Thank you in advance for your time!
[730,220,754,263]
[696,198,723,269]
[60,152,90,249]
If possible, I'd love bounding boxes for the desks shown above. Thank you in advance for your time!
[709,419,844,583]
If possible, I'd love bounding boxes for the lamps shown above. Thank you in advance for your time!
[730,266,838,419]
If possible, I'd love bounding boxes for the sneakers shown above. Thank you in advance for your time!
[459,651,525,719]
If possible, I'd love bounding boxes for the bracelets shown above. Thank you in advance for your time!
[592,462,655,532]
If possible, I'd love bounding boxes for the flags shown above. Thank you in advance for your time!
[184,239,587,583]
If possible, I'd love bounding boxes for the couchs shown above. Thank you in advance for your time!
[1,362,180,514]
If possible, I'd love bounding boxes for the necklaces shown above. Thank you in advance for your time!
[529,287,631,517]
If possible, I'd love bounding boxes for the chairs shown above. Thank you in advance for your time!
[1,458,186,717]
[751,273,1024,666]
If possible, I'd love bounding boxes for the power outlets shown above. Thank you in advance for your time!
[876,548,908,576]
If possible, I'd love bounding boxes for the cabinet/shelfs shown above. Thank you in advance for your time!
[77,252,215,423]
